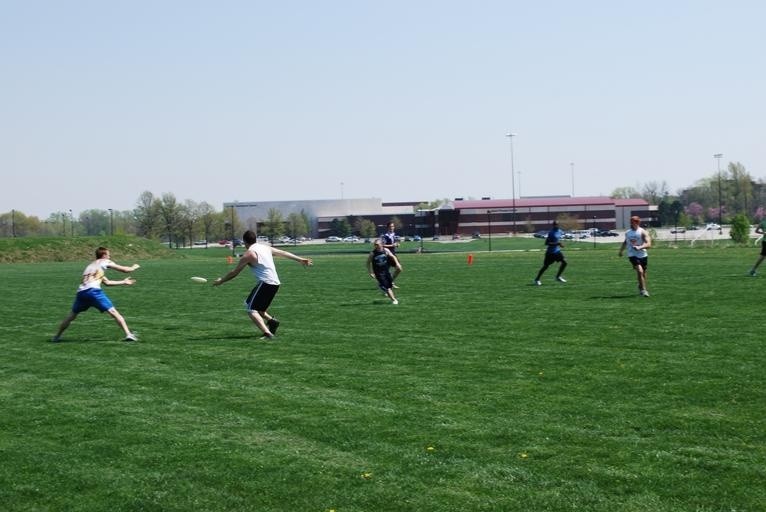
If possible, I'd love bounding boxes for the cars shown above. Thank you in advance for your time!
[472,231,481,238]
[532,227,620,239]
[255,236,312,244]
[706,223,722,230]
[326,235,362,242]
[414,235,421,240]
[231,239,241,245]
[451,232,462,240]
[219,240,228,244]
[671,227,685,233]
[433,234,441,241]
[160,242,176,247]
[404,236,412,241]
[686,224,699,230]
[365,239,372,243]
[393,236,400,242]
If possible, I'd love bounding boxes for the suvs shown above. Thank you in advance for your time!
[195,239,206,245]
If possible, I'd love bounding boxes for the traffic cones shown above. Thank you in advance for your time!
[226,256,233,264]
[467,254,473,265]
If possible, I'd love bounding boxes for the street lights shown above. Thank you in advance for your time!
[592,216,597,249]
[230,204,233,245]
[61,214,66,236]
[108,207,114,235]
[713,153,727,229]
[69,208,74,237]
[487,209,492,252]
[505,133,520,235]
[571,162,575,198]
[339,182,344,198]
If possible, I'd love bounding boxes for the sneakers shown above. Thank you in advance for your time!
[639,284,649,297]
[392,300,398,304]
[392,283,399,288]
[125,334,137,341]
[557,276,567,282]
[260,319,279,339]
[534,280,542,285]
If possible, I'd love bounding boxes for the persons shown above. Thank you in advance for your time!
[618,215,650,297]
[51,247,140,343]
[380,221,400,289]
[746,209,766,277]
[534,220,567,285]
[366,240,401,304]
[213,232,314,342]
